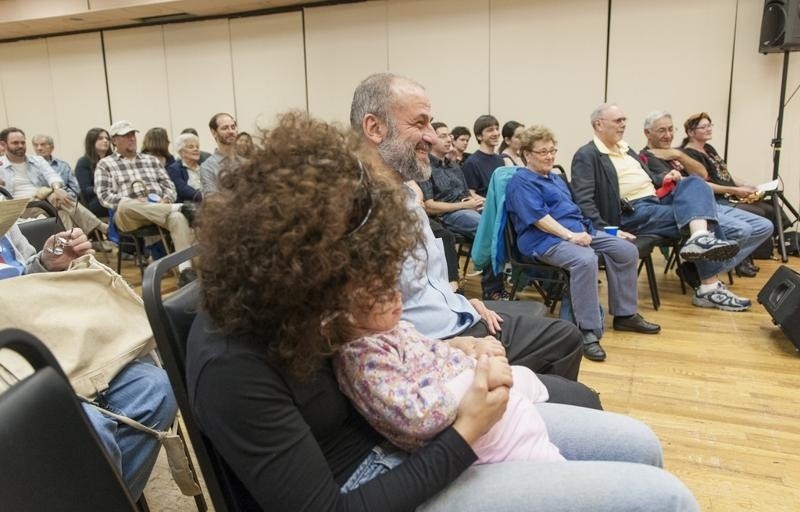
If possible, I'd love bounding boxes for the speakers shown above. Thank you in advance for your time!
[756,265,800,353]
[758,0,800,54]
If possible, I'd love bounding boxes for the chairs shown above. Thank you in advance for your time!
[453,164,687,315]
[0,328,137,508]
[142,241,258,508]
[18,203,180,281]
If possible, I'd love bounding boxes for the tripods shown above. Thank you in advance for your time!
[755,52,800,263]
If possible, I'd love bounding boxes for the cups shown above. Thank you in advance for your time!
[148,194,160,202]
[604,226,620,236]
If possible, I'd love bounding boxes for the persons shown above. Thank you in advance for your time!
[417,113,781,361]
[3,112,258,285]
[350,71,603,410]
[185,110,699,512]
[2,193,178,506]
[330,274,563,464]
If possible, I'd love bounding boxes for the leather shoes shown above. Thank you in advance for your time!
[180,201,198,227]
[177,267,198,287]
[583,330,605,360]
[613,313,660,334]
[735,255,759,278]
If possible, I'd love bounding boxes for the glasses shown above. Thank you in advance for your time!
[45,184,79,257]
[526,148,557,156]
[691,123,714,129]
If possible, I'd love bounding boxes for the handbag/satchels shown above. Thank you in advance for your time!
[0,253,158,404]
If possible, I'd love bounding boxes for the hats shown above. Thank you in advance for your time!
[109,121,140,139]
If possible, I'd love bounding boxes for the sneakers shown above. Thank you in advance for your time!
[91,241,112,252]
[675,261,701,290]
[679,231,741,262]
[504,260,536,289]
[482,285,520,301]
[692,281,751,311]
